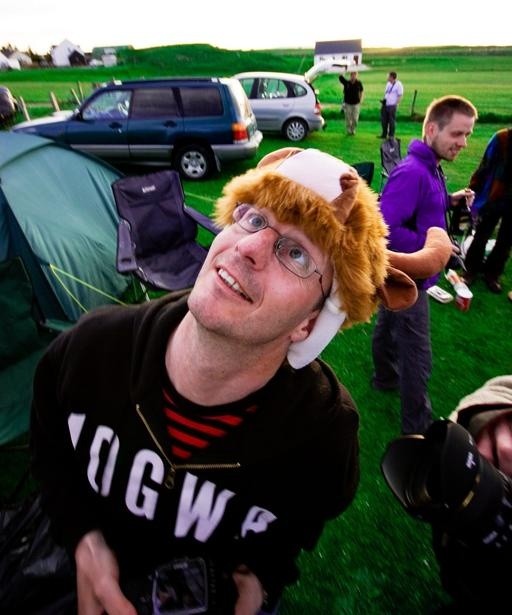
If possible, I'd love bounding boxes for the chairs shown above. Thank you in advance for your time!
[110,171,222,302]
[379,139,401,190]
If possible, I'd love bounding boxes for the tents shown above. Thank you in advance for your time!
[2,129,149,450]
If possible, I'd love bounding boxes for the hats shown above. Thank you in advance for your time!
[457,374,512,438]
[210,146,418,329]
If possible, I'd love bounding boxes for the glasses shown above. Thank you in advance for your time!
[231,200,325,299]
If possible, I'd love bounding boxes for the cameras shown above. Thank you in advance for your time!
[379,416,512,598]
[129,555,238,615]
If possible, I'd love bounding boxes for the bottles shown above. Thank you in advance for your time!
[451,274,472,298]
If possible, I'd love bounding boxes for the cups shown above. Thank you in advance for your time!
[454,289,472,312]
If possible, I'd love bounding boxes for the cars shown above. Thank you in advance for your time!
[233,62,371,140]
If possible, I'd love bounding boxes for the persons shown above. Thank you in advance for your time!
[28,145,420,615]
[368,94,480,437]
[376,71,405,141]
[336,67,365,138]
[432,373,512,615]
[461,128,510,296]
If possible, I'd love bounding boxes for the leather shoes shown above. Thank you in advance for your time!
[376,136,386,138]
[463,270,474,286]
[485,279,502,293]
[389,136,393,141]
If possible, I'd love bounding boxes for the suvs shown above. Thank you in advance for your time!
[10,78,262,180]
